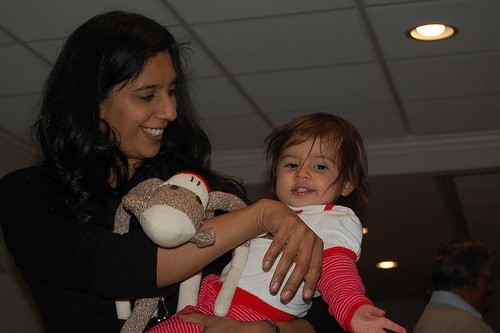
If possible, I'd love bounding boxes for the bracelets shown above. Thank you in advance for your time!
[263,318,280,333]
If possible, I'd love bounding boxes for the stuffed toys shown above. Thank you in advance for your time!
[114,170,253,331]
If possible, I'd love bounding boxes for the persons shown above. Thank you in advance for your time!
[384,238,456,332]
[5,9,346,332]
[412,249,500,332]
[144,111,407,333]
